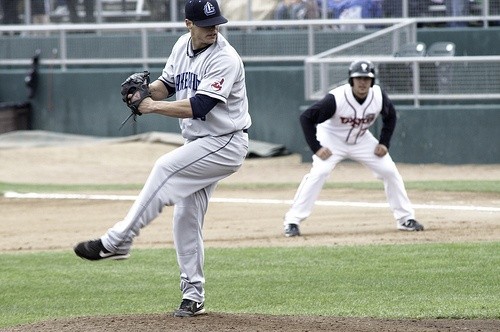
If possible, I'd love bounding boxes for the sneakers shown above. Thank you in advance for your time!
[175,297,205,318]
[74,239,130,262]
[400,218,422,231]
[285,223,298,237]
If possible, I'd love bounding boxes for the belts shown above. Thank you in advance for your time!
[243,129,247,133]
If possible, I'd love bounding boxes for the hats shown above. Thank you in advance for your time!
[186,0,228,27]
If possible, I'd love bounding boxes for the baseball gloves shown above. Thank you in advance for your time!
[120,70,153,116]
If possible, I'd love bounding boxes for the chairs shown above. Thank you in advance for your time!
[395,42,457,91]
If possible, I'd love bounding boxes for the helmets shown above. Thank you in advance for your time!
[348,61,375,88]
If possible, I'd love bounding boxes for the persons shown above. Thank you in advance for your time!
[76,1,253,316]
[284,60,425,236]
